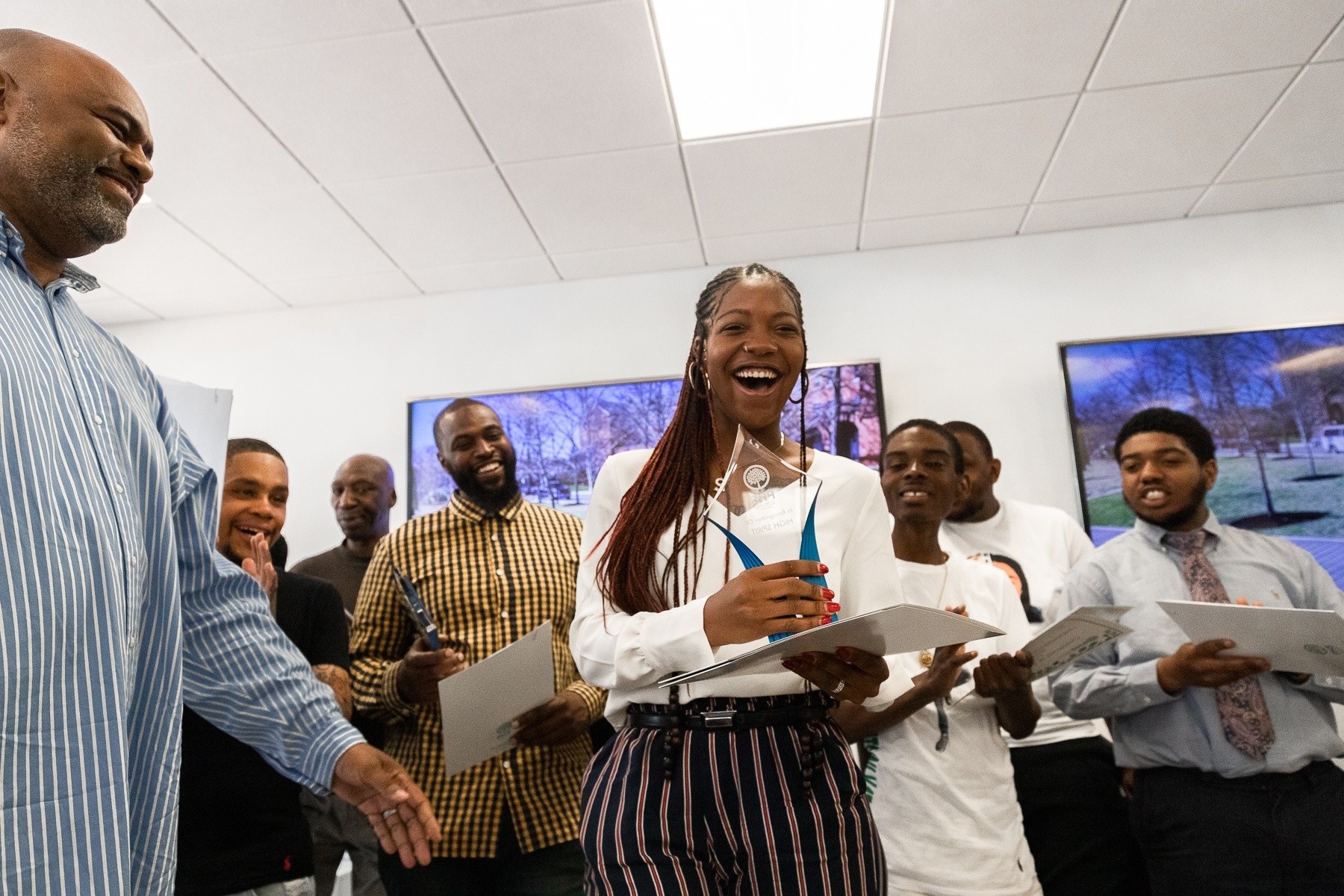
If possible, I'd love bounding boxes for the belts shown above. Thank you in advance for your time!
[631,705,829,729]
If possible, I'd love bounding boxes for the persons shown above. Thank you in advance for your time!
[173,399,607,896]
[0,28,439,896]
[826,421,1115,896]
[1047,408,1344,896]
[569,264,897,896]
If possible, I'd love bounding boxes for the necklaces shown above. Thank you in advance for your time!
[713,428,785,491]
[898,551,948,667]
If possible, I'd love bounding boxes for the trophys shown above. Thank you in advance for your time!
[700,424,838,644]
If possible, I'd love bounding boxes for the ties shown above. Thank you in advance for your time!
[1166,532,1276,762]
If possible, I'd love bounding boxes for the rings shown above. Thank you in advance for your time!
[383,809,398,820]
[832,680,844,693]
